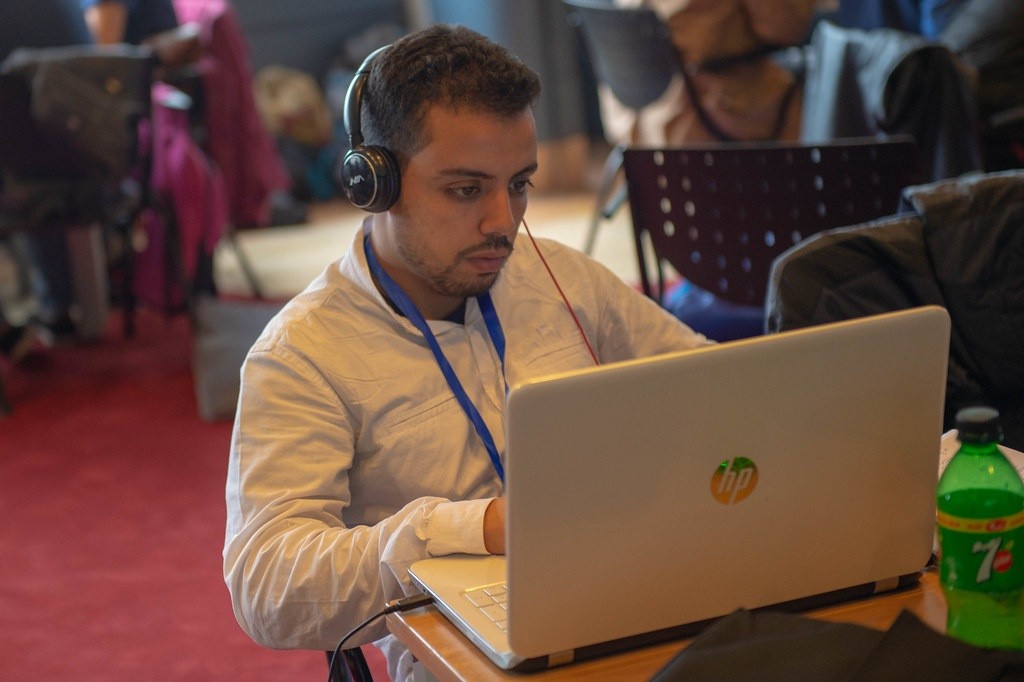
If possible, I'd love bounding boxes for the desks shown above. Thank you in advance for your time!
[382,425,1024,682]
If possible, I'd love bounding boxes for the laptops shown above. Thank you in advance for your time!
[408,306,953,672]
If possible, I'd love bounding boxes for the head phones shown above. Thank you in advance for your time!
[341,43,401,214]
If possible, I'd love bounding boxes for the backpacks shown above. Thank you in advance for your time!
[765,169,1024,452]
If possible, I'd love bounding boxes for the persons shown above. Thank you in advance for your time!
[220,24,725,682]
[0,1,308,375]
[612,0,808,144]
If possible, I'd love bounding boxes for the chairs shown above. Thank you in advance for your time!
[764,170,1024,453]
[166,0,266,301]
[560,0,812,306]
[622,134,916,344]
[0,41,151,339]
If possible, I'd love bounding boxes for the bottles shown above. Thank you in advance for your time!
[937,407,1024,649]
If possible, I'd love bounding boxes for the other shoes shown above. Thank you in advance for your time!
[0,310,79,356]
